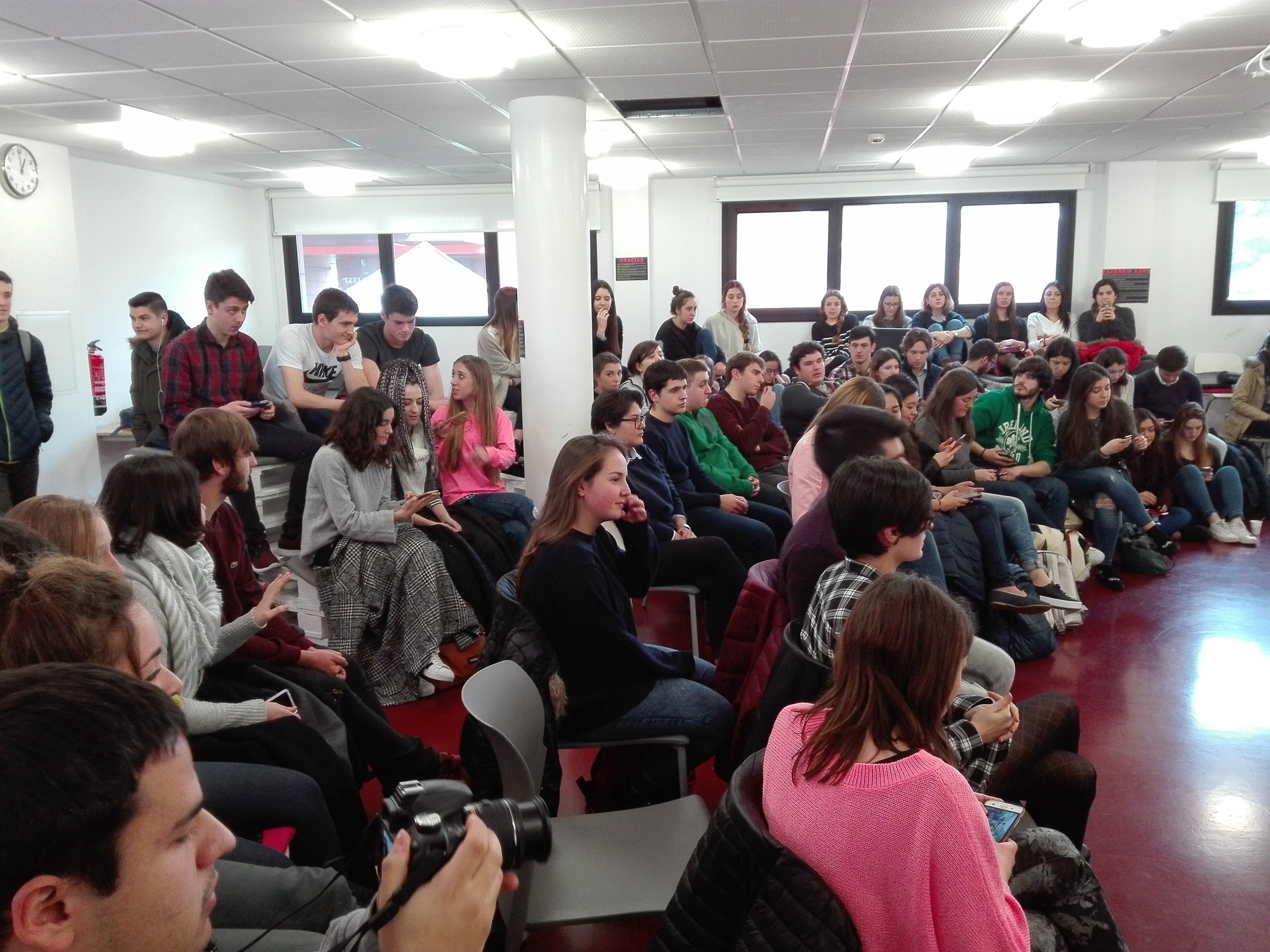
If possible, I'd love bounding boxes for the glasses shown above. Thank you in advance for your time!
[883,301,900,308]
[982,356,996,369]
[919,511,935,531]
[620,414,646,429]
[765,368,779,375]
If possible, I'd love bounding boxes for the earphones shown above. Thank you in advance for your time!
[162,319,164,326]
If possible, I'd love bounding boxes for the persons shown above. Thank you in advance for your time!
[0,269,1269,952]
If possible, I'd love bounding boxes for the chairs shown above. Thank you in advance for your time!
[652,744,860,952]
[455,658,712,952]
[777,479,793,517]
[714,552,782,719]
[758,613,838,761]
[497,568,692,798]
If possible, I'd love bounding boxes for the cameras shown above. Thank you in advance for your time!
[361,780,551,910]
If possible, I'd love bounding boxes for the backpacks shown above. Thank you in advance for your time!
[1033,549,1085,632]
[988,564,1057,662]
[1221,442,1270,518]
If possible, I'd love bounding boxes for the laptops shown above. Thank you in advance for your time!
[870,325,912,359]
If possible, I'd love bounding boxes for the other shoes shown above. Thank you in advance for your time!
[436,751,467,784]
[989,588,1051,613]
[1079,844,1090,863]
[1094,571,1125,590]
[1078,547,1105,565]
[1146,525,1177,555]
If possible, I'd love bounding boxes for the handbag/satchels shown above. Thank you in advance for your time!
[1054,526,1087,580]
[996,341,1025,355]
[1115,522,1167,573]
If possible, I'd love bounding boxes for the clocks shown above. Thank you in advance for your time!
[0,142,40,200]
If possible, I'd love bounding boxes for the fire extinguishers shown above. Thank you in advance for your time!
[86,339,107,417]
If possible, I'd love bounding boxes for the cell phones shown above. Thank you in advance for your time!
[951,434,966,451]
[998,453,1018,463]
[245,401,269,409]
[956,492,982,499]
[918,397,922,402]
[400,490,439,505]
[1103,301,1111,308]
[1146,508,1170,516]
[266,689,298,713]
[1125,434,1132,438]
[440,411,468,430]
[1200,466,1211,473]
[1162,420,1172,424]
[1052,399,1062,405]
[993,472,1008,476]
[983,800,1026,844]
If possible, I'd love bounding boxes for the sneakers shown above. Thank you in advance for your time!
[248,543,281,572]
[278,538,301,556]
[1227,519,1258,545]
[418,675,435,696]
[423,649,455,682]
[1034,583,1083,611]
[1209,518,1240,543]
